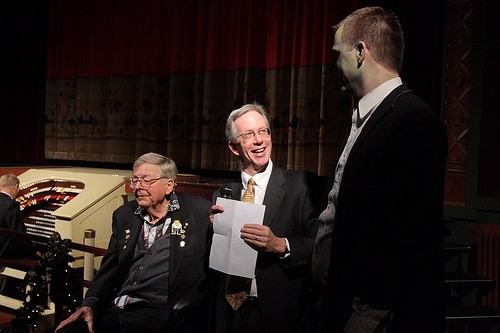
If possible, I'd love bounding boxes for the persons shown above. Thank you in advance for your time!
[0,174,41,265]
[209,104,331,333]
[312,7,446,332]
[55,152,213,333]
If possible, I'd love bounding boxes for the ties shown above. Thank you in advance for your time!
[242,178,255,203]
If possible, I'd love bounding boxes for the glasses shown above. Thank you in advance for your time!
[236,128,271,138]
[128,177,165,188]
